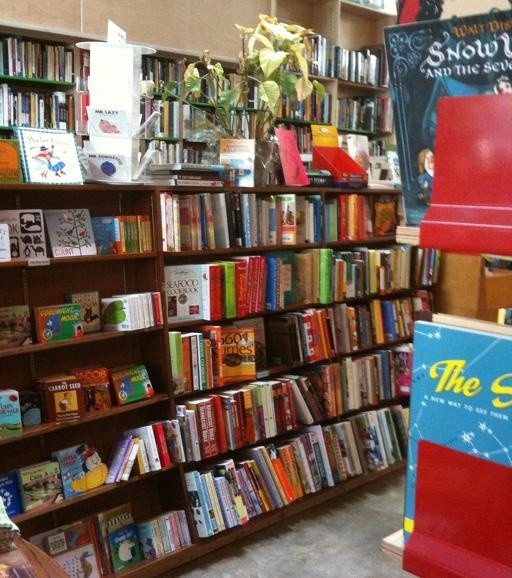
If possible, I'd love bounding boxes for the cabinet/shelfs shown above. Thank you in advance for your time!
[183,48,255,160]
[0,19,78,131]
[271,64,337,154]
[336,0,393,180]
[78,30,183,161]
[381,95,512,578]
[0,184,197,578]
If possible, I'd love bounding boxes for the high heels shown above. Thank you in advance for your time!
[61,171,66,175]
[55,173,61,177]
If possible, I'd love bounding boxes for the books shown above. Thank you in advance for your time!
[383,10,512,226]
[403,255,512,539]
[0,36,434,578]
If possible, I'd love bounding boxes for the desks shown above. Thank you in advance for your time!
[158,185,441,559]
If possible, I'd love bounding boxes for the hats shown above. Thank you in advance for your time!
[40,146,48,151]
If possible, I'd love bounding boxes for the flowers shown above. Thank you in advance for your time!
[141,14,329,141]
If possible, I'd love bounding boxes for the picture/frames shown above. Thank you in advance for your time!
[19,126,84,186]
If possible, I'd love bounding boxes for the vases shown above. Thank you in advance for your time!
[256,140,277,189]
